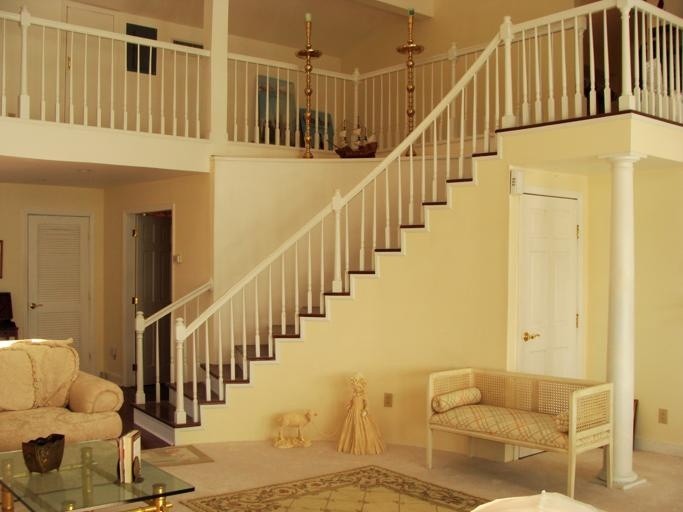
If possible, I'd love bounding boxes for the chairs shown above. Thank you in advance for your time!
[0,370,125,452]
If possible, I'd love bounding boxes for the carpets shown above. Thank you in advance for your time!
[176,462,491,512]
[140,445,214,468]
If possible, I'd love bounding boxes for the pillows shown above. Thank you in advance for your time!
[432,386,482,413]
[555,409,569,432]
[0,338,80,411]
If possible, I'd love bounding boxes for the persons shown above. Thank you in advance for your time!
[335,374,385,455]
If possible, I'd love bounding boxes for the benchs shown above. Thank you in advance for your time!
[426,367,614,499]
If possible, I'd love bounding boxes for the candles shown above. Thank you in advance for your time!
[407,6,415,16]
[304,11,313,21]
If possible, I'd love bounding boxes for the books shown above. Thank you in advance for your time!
[116,428,142,484]
